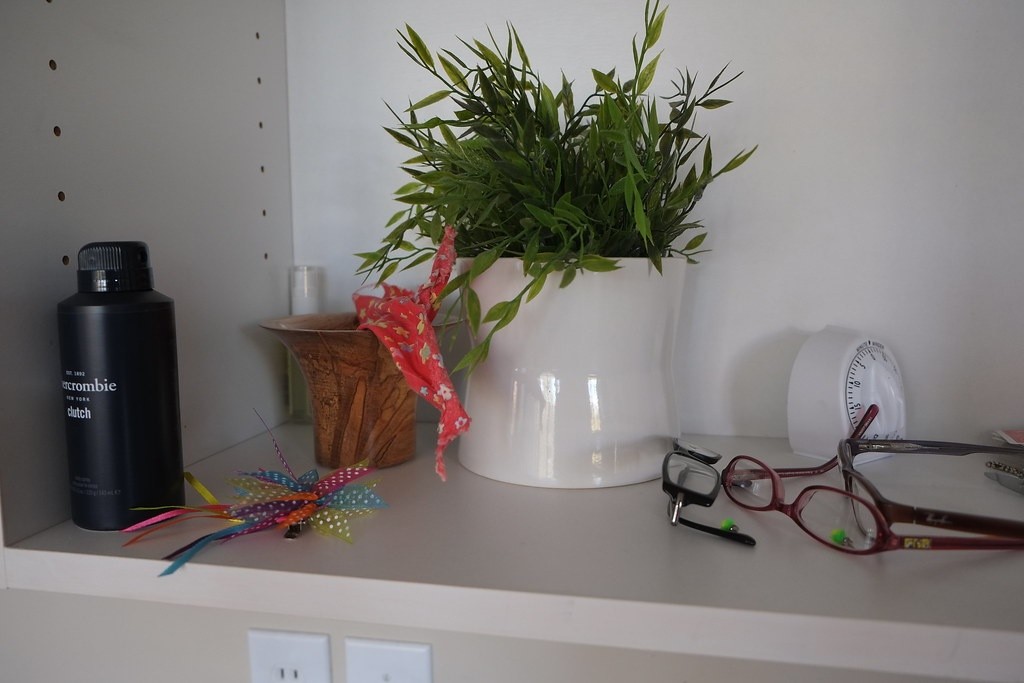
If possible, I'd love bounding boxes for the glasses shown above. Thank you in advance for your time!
[836,437,1024,541]
[662,437,759,547]
[721,405,1024,557]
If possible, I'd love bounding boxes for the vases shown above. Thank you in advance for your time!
[262,309,466,469]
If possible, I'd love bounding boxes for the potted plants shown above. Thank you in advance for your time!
[356,0,761,495]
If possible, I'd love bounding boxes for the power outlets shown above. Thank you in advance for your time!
[344,637,432,683]
[247,628,332,683]
